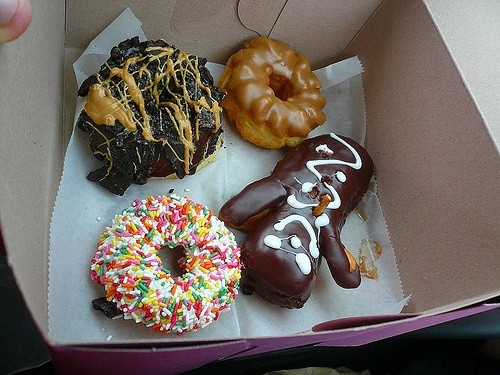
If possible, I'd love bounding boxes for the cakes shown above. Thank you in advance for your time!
[218,134,375,311]
[76,37,228,196]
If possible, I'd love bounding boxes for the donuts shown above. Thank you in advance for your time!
[90,195,244,334]
[217,35,326,151]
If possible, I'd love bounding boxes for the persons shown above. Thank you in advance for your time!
[0,0,33,45]
